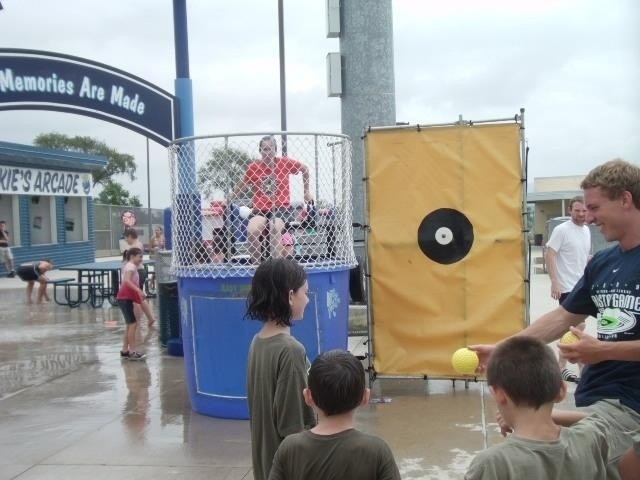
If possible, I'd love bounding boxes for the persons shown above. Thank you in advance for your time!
[0,221,16,277]
[241,258,312,479]
[269,350,402,479]
[464,157,640,480]
[222,136,313,265]
[149,226,165,289]
[116,248,147,360]
[18,259,53,304]
[119,229,157,328]
[545,196,592,382]
[464,334,609,480]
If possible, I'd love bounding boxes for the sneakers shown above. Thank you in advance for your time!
[120,351,128,356]
[127,352,145,360]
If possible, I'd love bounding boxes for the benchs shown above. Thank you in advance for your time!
[225,198,339,259]
[45,270,157,308]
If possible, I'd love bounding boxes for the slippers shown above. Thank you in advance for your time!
[561,369,577,380]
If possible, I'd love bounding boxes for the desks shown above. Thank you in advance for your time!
[58,259,156,303]
